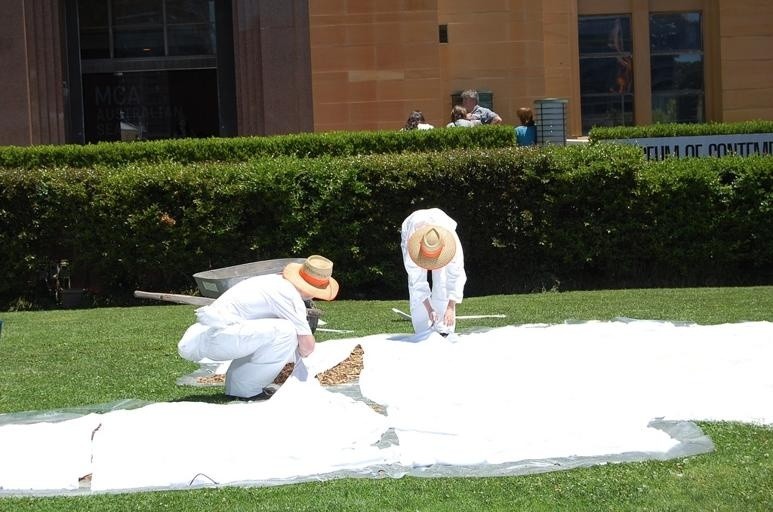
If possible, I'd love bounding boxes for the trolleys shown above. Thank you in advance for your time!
[134,257,323,334]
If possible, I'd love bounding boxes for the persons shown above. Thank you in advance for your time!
[460,88,502,124]
[403,109,434,129]
[513,107,536,146]
[400,207,467,335]
[446,105,474,128]
[177,254,340,402]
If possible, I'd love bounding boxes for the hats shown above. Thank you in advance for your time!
[282,255,340,301]
[407,225,456,269]
